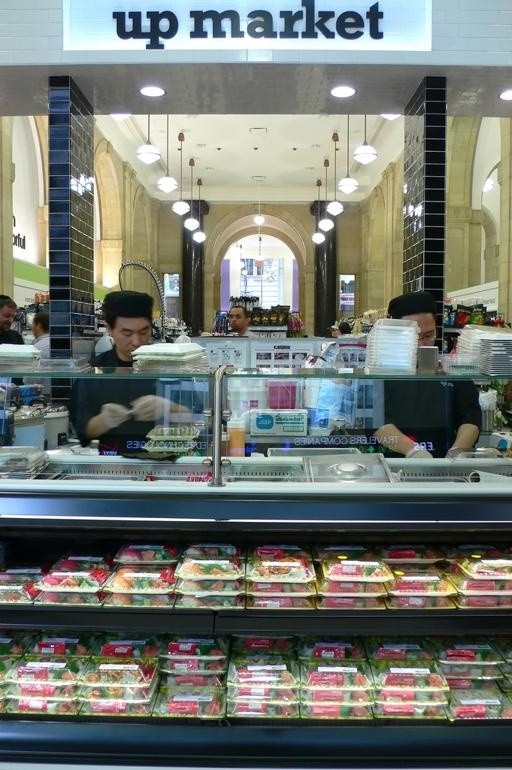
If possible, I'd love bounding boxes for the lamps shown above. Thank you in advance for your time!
[135,113,378,244]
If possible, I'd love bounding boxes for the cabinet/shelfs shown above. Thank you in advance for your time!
[0,325,512,769]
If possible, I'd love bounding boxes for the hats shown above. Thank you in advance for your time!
[102,289,155,319]
[387,290,440,320]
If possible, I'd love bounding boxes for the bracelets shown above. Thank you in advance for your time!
[405,443,424,458]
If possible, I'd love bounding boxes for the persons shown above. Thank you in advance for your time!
[339,320,353,338]
[227,305,258,338]
[22,310,52,402]
[1,293,27,387]
[356,291,482,460]
[68,291,207,457]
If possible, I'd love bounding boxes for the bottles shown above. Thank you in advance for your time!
[227,413,245,456]
